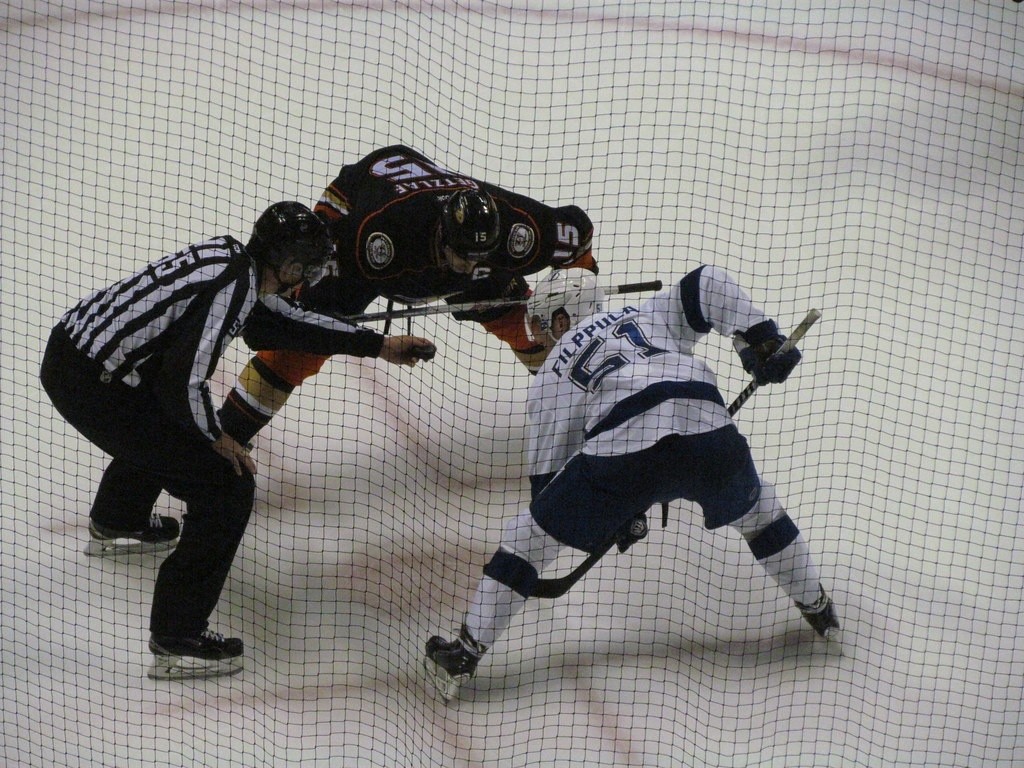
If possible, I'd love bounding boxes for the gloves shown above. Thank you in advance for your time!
[733,318,803,386]
[616,514,647,553]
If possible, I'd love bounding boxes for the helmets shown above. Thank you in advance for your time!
[443,189,500,263]
[528,267,603,331]
[252,202,332,273]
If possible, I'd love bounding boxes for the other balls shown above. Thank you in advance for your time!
[411,345,436,360]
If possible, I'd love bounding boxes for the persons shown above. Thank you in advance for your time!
[42,202,437,680]
[425,263,847,700]
[218,144,599,452]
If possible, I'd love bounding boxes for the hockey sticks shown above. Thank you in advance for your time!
[480,307,823,599]
[341,279,664,323]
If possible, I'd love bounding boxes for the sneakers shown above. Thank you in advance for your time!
[422,612,488,700]
[148,629,243,679]
[83,513,180,556]
[795,581,844,646]
